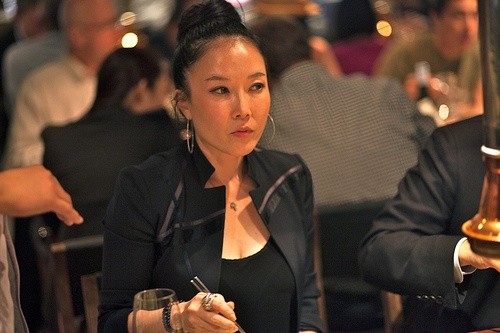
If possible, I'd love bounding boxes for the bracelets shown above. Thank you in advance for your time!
[161,300,183,333]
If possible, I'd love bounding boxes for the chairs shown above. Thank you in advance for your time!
[42,194,405,333]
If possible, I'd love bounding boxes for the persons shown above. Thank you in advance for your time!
[0,0,500,332]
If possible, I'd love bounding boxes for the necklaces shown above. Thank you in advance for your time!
[229,202,238,213]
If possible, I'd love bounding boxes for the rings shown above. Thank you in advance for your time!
[200,289,217,311]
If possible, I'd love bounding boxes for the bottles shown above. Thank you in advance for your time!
[411,61,444,128]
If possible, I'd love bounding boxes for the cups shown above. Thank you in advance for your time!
[133,288,184,333]
